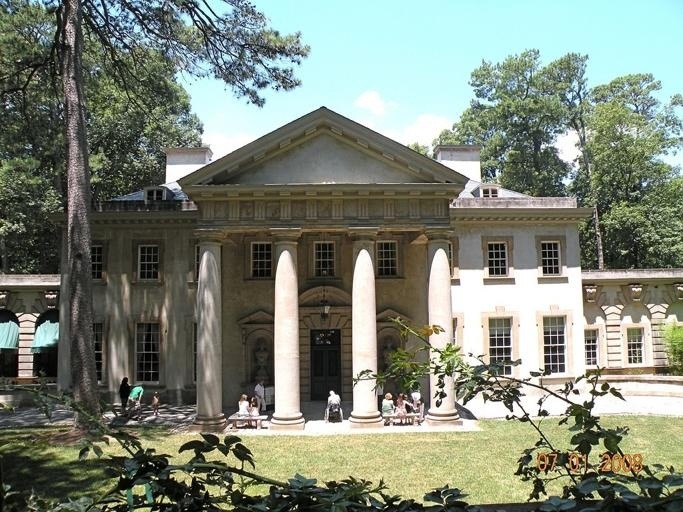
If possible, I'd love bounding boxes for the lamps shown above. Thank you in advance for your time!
[318,287,331,322]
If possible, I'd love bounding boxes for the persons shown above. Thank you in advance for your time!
[324,389,344,422]
[152,391,161,417]
[126,384,148,421]
[377,383,425,422]
[238,379,267,427]
[118,376,131,414]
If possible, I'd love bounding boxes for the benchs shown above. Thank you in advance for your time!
[227,414,267,430]
[381,412,421,426]
